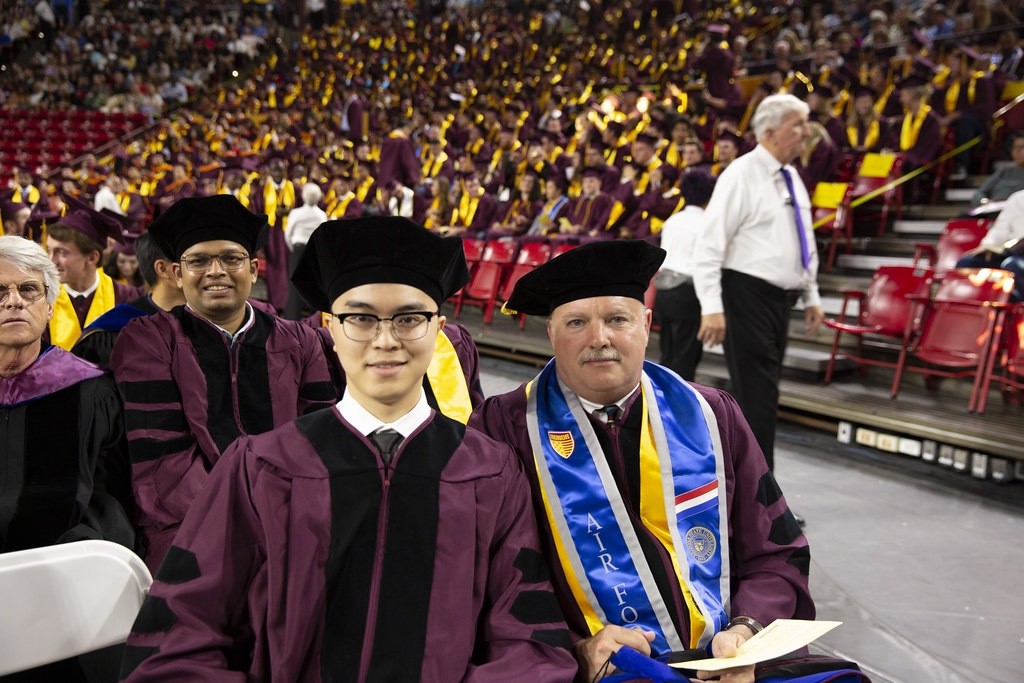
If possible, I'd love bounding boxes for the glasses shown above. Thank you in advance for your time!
[0,281,49,303]
[180,254,248,271]
[331,309,440,341]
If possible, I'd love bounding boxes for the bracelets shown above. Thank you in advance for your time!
[724,616,764,634]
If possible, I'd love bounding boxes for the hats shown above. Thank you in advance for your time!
[292,215,473,312]
[55,191,125,250]
[506,241,667,316]
[956,44,982,64]
[913,29,928,44]
[917,57,938,77]
[149,193,267,263]
[0,189,28,219]
[707,24,729,37]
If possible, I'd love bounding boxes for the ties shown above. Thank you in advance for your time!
[74,295,86,306]
[597,405,621,430]
[778,167,809,267]
[367,431,402,472]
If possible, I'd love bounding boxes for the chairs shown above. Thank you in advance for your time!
[0,106,152,190]
[812,153,1024,416]
[449,236,661,331]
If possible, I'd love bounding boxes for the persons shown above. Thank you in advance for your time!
[0,236,138,683]
[687,94,823,473]
[0,0,1024,383]
[466,240,872,683]
[111,195,486,683]
